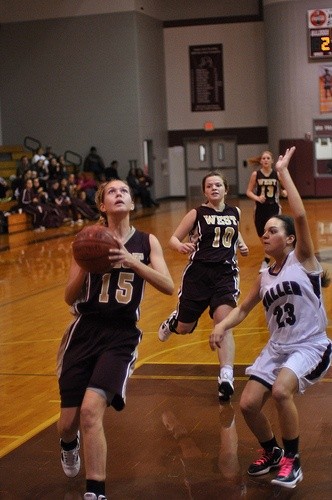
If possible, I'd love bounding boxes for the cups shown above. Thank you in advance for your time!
[18,208,22,214]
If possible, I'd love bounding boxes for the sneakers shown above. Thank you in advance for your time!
[162,410,180,435]
[84,492,106,500]
[60,429,81,477]
[218,373,234,399]
[247,448,284,475]
[271,454,303,489]
[159,310,179,342]
[218,398,235,430]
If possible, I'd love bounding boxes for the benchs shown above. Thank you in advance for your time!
[0,144,98,234]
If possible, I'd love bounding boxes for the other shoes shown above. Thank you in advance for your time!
[34,226,45,232]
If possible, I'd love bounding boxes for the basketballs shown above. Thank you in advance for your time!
[72,225,120,275]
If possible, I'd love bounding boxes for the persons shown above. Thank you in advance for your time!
[158,171,248,401]
[56,178,175,500]
[246,150,287,274]
[209,147,332,488]
[320,69,332,98]
[0,146,160,228]
